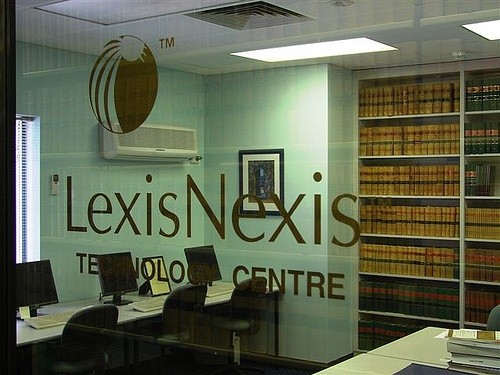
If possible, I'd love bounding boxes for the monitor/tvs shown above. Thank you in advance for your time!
[15,259,59,320]
[184,245,222,286]
[97,252,138,306]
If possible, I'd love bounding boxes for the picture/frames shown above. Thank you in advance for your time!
[238,148,286,216]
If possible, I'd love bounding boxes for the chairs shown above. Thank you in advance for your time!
[203,276,269,372]
[44,303,120,375]
[131,283,208,373]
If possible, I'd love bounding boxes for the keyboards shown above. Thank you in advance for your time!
[128,296,167,313]
[25,310,78,329]
[206,284,234,298]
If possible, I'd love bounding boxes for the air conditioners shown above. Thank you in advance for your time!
[97,120,202,164]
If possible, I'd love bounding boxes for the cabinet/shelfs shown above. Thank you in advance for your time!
[354,56,500,357]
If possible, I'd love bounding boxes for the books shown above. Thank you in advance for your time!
[358,76,500,375]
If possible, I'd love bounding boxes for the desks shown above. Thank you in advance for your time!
[311,326,500,375]
[17,281,282,375]
